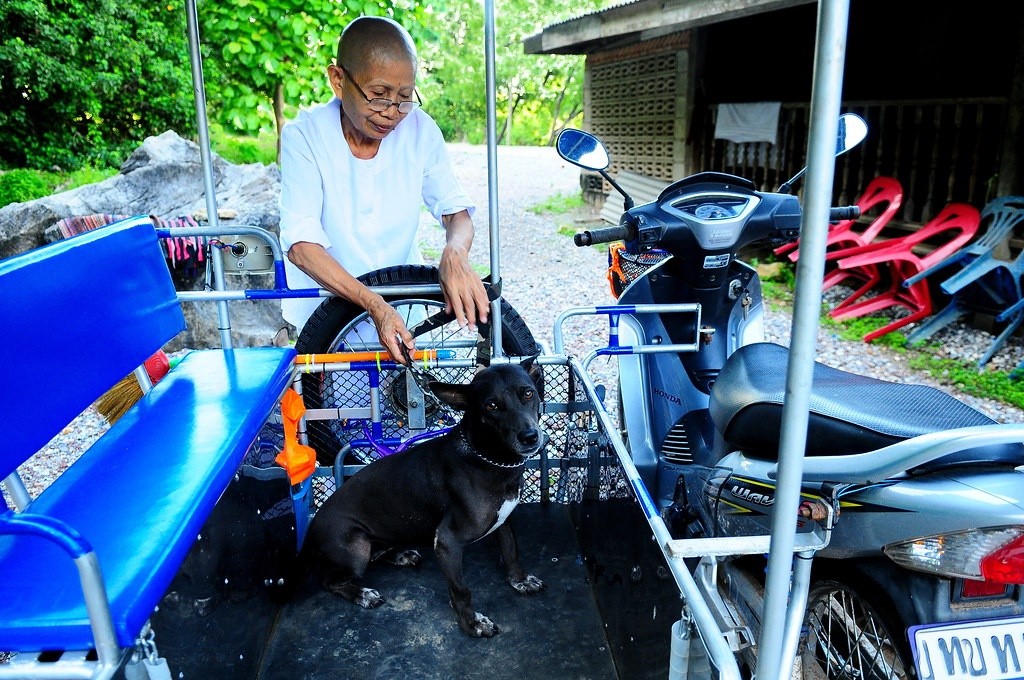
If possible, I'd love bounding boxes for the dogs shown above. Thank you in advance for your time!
[266,354,548,638]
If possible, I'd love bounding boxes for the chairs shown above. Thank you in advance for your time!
[776,176,904,316]
[904,197,1024,368]
[822,202,982,346]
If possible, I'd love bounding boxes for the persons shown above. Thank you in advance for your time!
[277,16,491,365]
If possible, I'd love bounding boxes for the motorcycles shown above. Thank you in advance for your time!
[554,112,1024,680]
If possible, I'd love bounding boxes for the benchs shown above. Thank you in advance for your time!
[0,213,300,680]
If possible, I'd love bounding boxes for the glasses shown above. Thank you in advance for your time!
[337,63,423,114]
